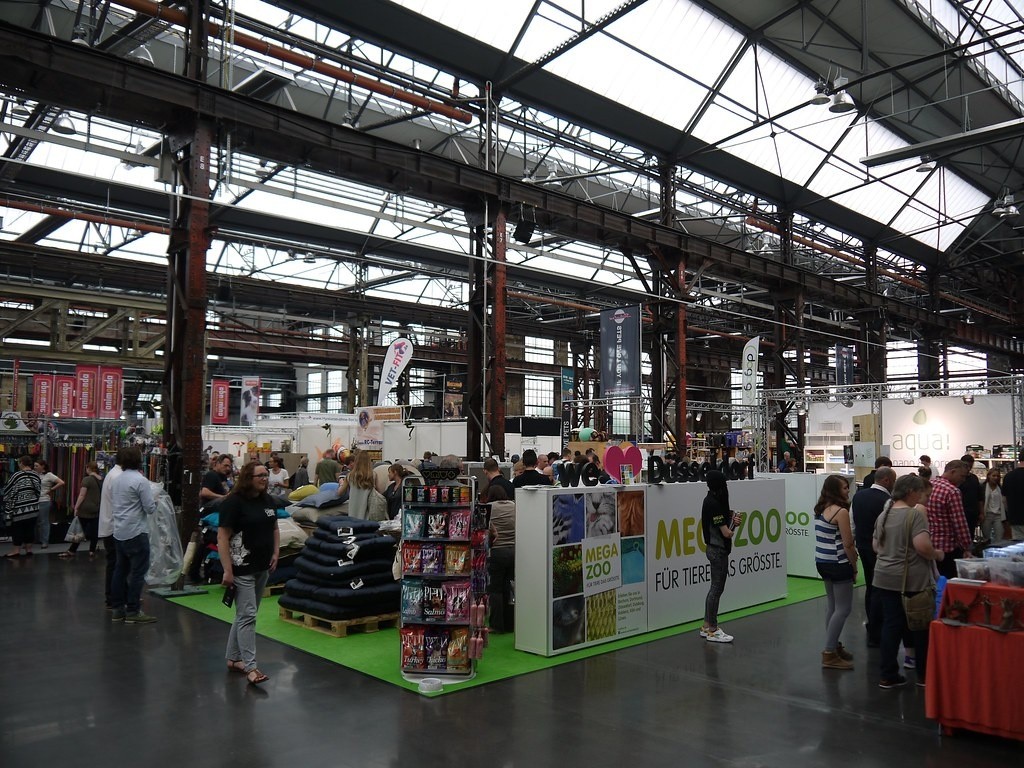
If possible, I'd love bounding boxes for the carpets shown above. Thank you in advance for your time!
[0,537,106,557]
[164,555,866,699]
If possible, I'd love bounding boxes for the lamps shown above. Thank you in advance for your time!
[72,30,89,46]
[686,410,702,421]
[544,163,561,189]
[962,395,974,405]
[52,112,76,134]
[992,188,1021,218]
[758,237,773,255]
[811,66,855,113]
[129,44,153,64]
[11,103,29,115]
[904,392,914,404]
[720,412,745,422]
[841,396,853,407]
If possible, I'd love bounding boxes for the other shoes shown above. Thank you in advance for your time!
[5,553,20,558]
[707,628,733,642]
[916,680,925,686]
[112,609,125,621]
[837,642,850,658]
[125,612,158,623]
[700,627,709,636]
[879,675,908,688]
[105,606,112,610]
[41,544,48,548]
[58,550,76,556]
[26,552,33,556]
[822,651,853,669]
[89,551,95,557]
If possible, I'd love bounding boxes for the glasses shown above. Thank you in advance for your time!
[253,474,268,477]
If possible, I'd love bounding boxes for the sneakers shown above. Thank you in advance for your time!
[903,656,916,668]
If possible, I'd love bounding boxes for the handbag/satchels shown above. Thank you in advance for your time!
[64,516,87,543]
[289,473,295,488]
[902,587,933,631]
[1001,521,1012,539]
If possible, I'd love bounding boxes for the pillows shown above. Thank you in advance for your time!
[200,482,349,560]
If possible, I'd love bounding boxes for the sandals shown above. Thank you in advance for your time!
[246,669,269,684]
[228,660,247,673]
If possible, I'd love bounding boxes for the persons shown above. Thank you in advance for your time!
[778,451,798,472]
[749,448,752,453]
[338,451,377,520]
[419,452,437,469]
[381,465,409,520]
[292,457,310,491]
[218,460,280,683]
[0,456,41,560]
[203,445,290,496]
[510,448,601,487]
[97,448,158,622]
[732,451,744,469]
[314,449,341,488]
[32,459,64,548]
[700,471,742,642]
[171,454,235,590]
[482,458,515,501]
[58,461,104,558]
[814,449,1024,688]
[487,485,513,633]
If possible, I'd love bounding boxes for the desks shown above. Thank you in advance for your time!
[925,579,1024,742]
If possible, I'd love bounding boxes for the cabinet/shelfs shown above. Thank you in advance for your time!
[803,433,853,474]
[400,477,478,683]
[973,459,1019,479]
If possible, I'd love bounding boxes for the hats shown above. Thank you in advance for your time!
[1018,448,1024,455]
[706,470,728,487]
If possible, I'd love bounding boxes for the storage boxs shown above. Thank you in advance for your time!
[954,557,1024,587]
